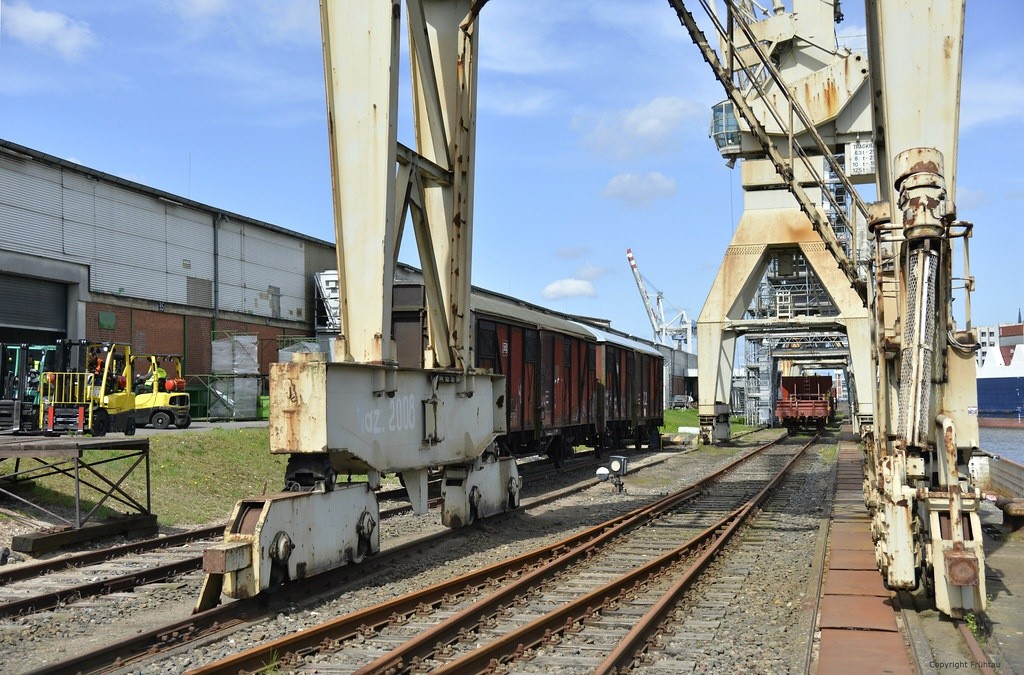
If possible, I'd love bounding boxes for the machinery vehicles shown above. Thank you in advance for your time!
[126,353,191,429]
[0,331,134,440]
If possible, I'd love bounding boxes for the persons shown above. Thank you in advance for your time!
[88,351,113,393]
[28,355,48,396]
[135,362,167,397]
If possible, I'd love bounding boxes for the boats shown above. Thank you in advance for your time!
[975,309,1024,419]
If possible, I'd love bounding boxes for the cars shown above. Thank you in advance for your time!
[671,395,688,408]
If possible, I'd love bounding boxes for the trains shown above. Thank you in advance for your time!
[771,374,839,435]
[391,262,665,488]
